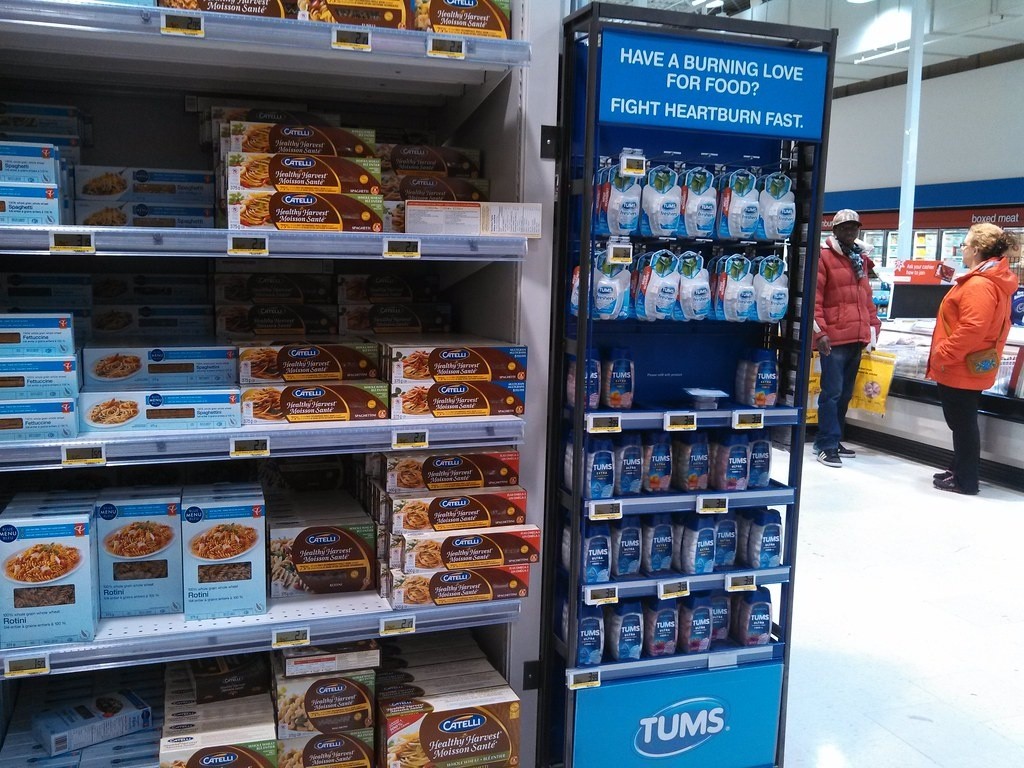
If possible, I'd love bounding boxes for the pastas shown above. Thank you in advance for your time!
[82,173,128,226]
[7,543,79,581]
[96,352,140,377]
[241,347,284,419]
[194,523,256,558]
[375,144,407,233]
[395,460,443,605]
[401,351,435,415]
[269,536,301,590]
[394,738,430,768]
[91,397,138,424]
[105,520,172,556]
[240,125,275,226]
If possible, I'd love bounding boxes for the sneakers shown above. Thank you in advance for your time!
[813,441,856,458]
[817,448,842,466]
[933,476,979,495]
[934,470,953,480]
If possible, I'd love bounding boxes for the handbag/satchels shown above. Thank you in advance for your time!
[965,348,999,374]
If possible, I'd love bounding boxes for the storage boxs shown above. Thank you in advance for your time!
[0,254,528,441]
[0,450,541,651]
[103,0,514,39]
[0,630,523,768]
[1,90,492,235]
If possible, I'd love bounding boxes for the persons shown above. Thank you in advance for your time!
[926,222,1019,495]
[813,208,881,467]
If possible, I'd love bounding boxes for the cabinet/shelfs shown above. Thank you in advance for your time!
[0,0,544,768]
[537,2,839,767]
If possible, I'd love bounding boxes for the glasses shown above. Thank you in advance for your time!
[960,243,979,252]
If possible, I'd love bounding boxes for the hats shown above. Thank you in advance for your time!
[832,209,862,229]
